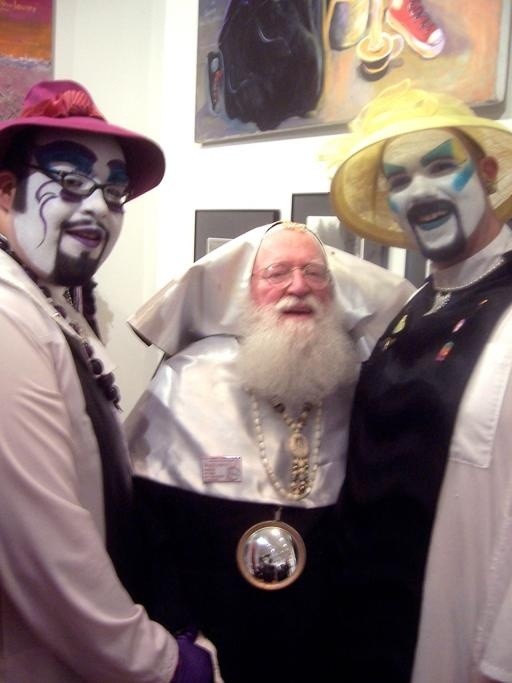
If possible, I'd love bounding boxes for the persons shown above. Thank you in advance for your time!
[128,218,418,683]
[3,77,216,682]
[326,79,510,682]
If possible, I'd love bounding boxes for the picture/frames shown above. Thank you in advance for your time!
[190,190,388,268]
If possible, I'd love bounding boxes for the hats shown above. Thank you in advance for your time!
[330,77,512,250]
[0,79,165,204]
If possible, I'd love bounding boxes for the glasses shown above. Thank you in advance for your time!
[253,262,331,291]
[25,163,131,209]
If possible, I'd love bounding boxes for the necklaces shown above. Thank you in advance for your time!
[250,381,323,502]
[272,395,311,493]
